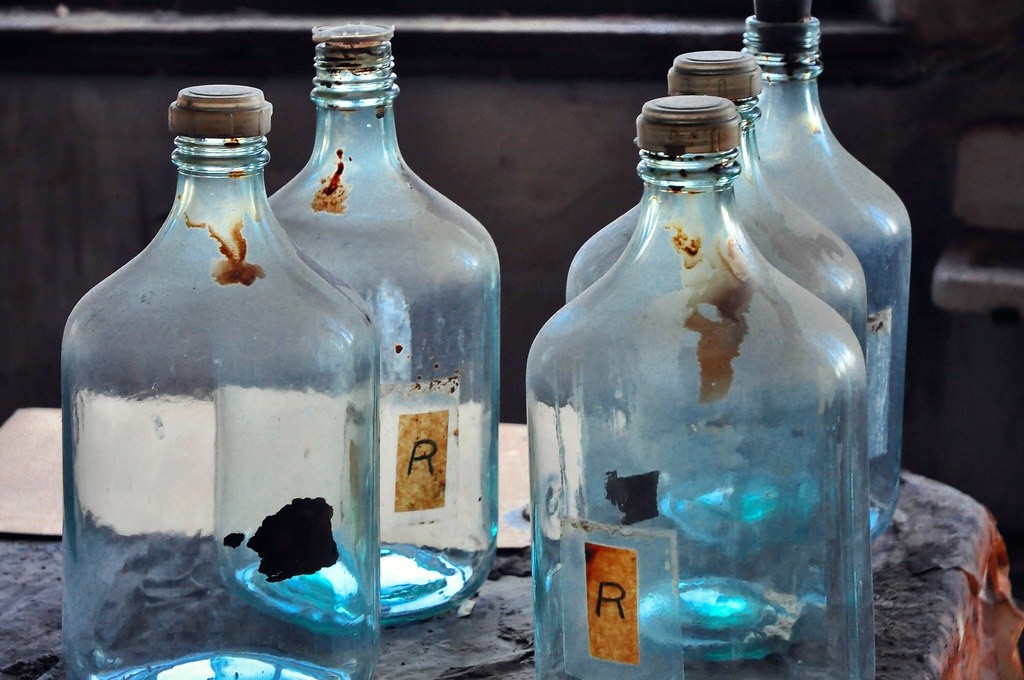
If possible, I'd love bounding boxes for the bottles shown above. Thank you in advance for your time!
[526,94,878,680]
[59,82,380,677]
[732,1,912,540]
[564,49,867,660]
[214,19,502,626]
